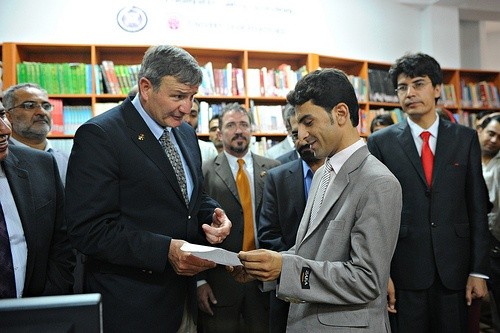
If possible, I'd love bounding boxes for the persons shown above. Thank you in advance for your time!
[65,45,232,333]
[197,104,282,333]
[257,102,327,333]
[475,112,500,333]
[0,98,76,333]
[370,114,394,134]
[367,53,491,333]
[227,68,403,333]
[2,83,70,187]
[183,99,223,164]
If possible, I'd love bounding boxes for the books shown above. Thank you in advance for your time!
[51,100,93,136]
[349,70,500,135]
[250,136,279,157]
[197,100,288,134]
[16,60,93,95]
[95,60,309,97]
[96,101,124,116]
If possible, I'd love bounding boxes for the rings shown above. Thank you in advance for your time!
[217,237,222,243]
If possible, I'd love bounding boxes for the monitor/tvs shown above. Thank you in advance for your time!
[0,293,103,333]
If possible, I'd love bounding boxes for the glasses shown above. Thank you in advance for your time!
[8,100,54,113]
[290,133,298,141]
[395,80,432,96]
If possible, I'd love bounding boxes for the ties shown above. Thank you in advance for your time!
[307,160,332,231]
[419,132,435,188]
[159,128,190,208]
[0,202,18,299]
[234,159,257,252]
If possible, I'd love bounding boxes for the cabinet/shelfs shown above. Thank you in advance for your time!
[0,42,500,142]
